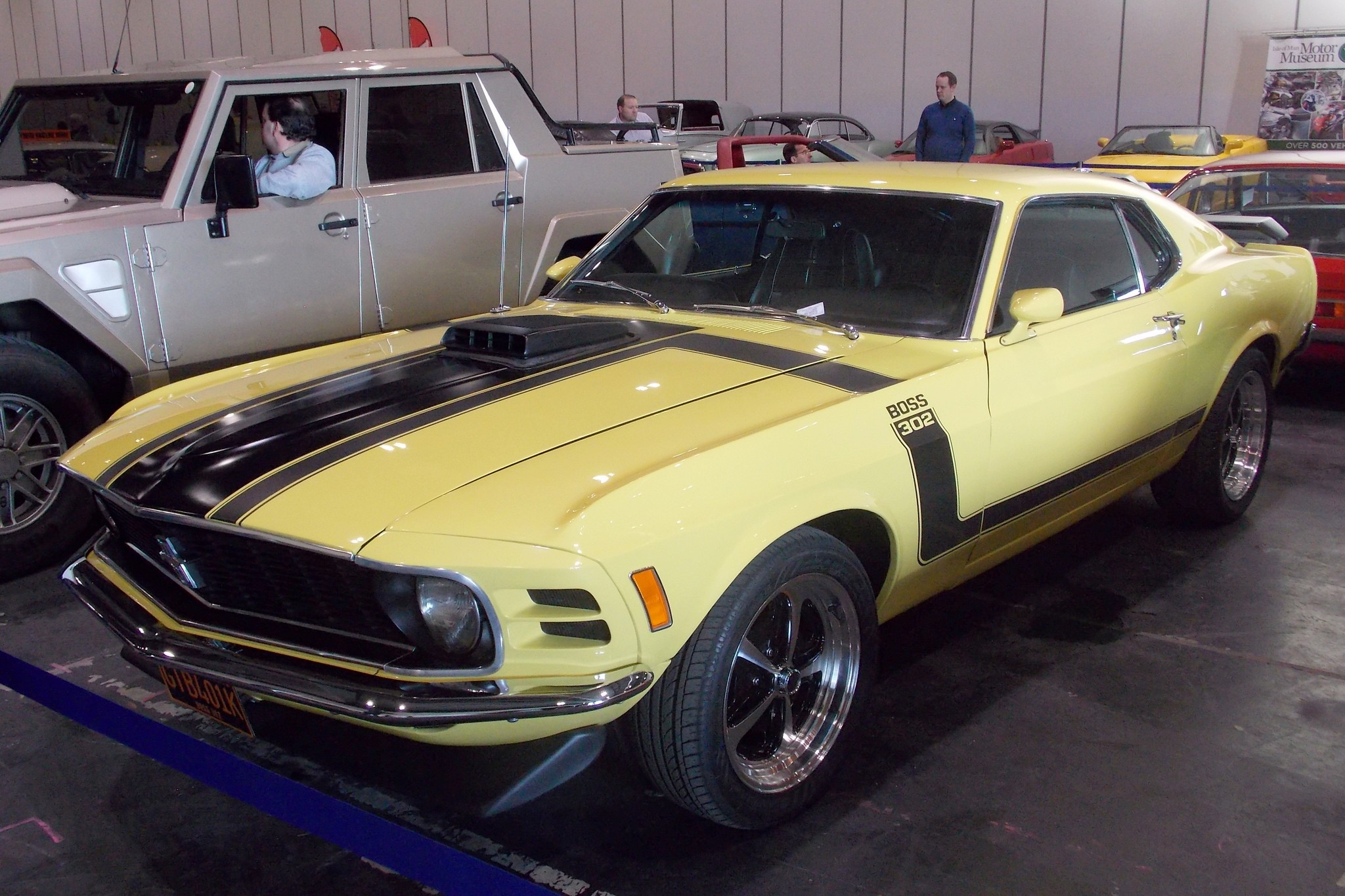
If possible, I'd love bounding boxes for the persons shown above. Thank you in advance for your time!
[240,95,336,202]
[783,142,813,164]
[915,71,975,162]
[603,93,661,141]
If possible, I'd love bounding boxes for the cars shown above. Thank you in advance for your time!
[1163,150,1345,369]
[881,120,1055,164]
[56,162,1320,833]
[680,111,905,171]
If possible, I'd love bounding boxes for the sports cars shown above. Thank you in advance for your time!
[637,98,756,151]
[1082,123,1268,214]
[717,134,888,169]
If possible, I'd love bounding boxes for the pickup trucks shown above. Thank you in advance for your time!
[0,44,696,584]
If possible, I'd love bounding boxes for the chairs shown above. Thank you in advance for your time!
[748,227,874,321]
